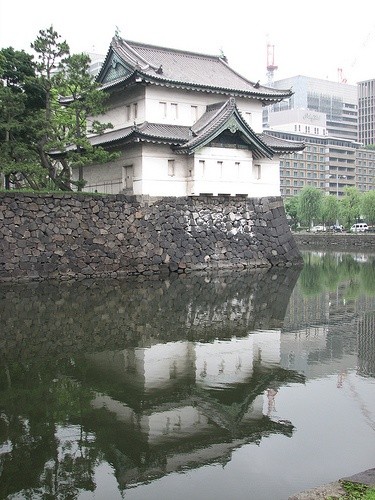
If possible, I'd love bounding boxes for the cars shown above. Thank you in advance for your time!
[310,225,343,232]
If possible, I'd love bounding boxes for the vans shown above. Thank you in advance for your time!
[349,223,368,233]
